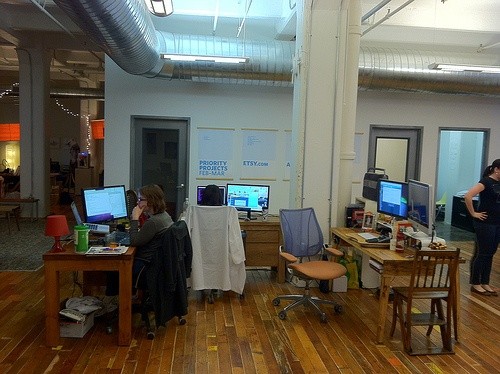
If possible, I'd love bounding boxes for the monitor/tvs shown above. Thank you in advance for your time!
[225,184,270,220]
[81,185,129,223]
[197,186,225,205]
[376,179,437,244]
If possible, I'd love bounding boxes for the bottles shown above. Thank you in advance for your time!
[396,229,404,253]
[74,225,90,254]
[262,207,268,220]
[347,217,352,226]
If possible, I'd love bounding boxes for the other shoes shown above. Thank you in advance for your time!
[95,298,117,318]
[482,285,497,296]
[471,286,491,296]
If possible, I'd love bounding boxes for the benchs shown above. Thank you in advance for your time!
[0,197,40,224]
[0,205,22,235]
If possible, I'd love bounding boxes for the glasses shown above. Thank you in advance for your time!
[137,199,147,202]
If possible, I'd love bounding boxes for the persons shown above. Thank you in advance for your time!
[465,159,500,295]
[200,185,222,206]
[105,184,174,296]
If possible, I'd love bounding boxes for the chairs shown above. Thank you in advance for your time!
[389,245,461,357]
[272,206,346,325]
[186,204,249,304]
[105,220,189,339]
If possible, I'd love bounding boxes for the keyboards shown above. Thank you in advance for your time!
[358,233,377,240]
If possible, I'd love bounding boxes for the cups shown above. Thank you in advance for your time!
[390,239,396,251]
[105,233,115,246]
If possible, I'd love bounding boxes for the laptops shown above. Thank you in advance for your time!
[71,201,109,234]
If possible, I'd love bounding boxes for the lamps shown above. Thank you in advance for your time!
[43,214,70,253]
[144,0,250,64]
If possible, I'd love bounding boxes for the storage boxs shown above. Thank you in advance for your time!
[60,311,96,338]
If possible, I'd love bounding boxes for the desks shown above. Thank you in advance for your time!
[238,214,285,285]
[39,237,137,349]
[328,225,467,346]
